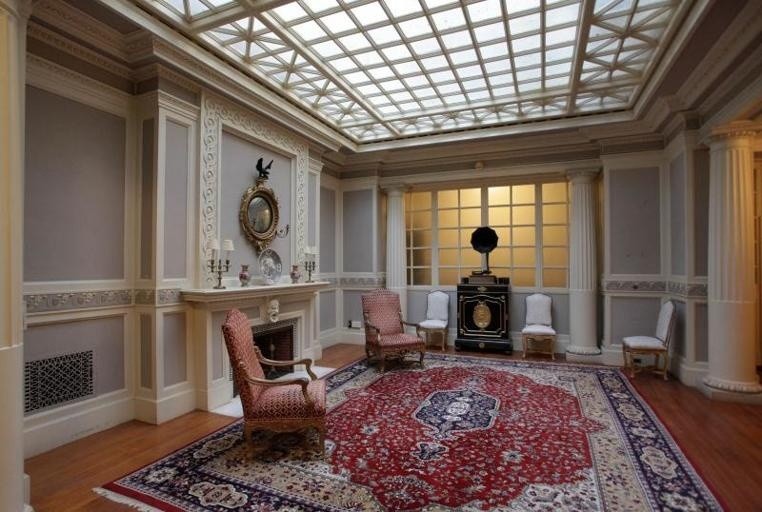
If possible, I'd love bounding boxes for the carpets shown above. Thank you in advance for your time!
[91,351,733,512]
[210,365,336,419]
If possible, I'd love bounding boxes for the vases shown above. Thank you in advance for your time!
[238,264,250,286]
[291,264,300,282]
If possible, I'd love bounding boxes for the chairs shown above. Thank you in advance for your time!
[621,300,675,381]
[522,293,556,360]
[418,291,450,352]
[362,288,427,373]
[221,308,327,457]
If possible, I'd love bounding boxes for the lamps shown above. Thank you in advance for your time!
[304,245,319,283]
[207,239,233,289]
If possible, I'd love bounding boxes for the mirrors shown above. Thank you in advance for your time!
[238,157,281,254]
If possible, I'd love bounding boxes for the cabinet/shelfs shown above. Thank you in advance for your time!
[455,278,513,355]
